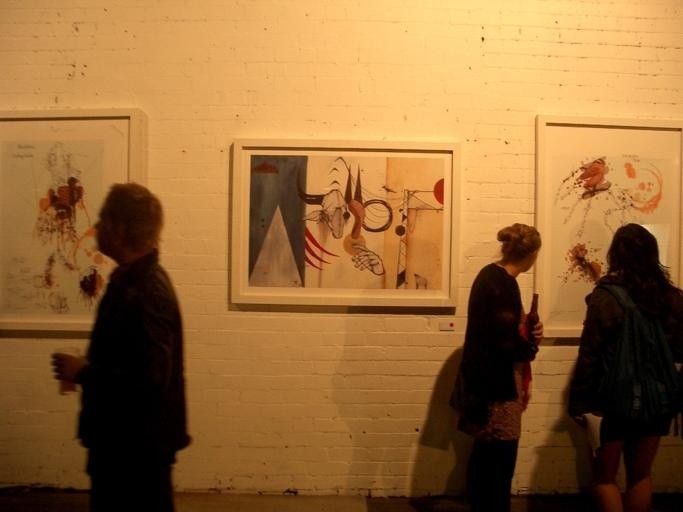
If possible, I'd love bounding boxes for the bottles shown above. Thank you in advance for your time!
[529,294,540,342]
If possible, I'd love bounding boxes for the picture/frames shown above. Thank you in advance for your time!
[532,114,683,338]
[0,108,148,333]
[231,138,460,309]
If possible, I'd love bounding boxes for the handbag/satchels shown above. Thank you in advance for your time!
[596,307,681,427]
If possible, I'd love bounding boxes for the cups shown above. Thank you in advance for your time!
[54,346,79,395]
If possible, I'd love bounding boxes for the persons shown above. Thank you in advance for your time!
[565,221,681,512]
[48,181,193,511]
[448,221,545,511]
